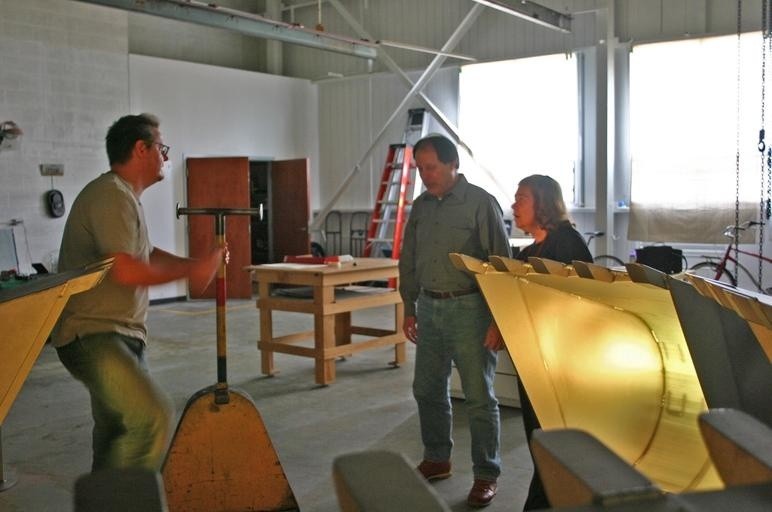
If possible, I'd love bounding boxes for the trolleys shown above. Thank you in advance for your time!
[349,211,370,287]
[322,211,344,289]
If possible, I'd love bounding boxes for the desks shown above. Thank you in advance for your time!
[240,254,409,385]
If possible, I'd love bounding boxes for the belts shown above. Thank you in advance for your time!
[419,288,481,300]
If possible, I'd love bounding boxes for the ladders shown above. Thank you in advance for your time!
[358,143,412,291]
[371,107,431,258]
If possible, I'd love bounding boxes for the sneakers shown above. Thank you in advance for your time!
[468,478,497,506]
[417,460,451,481]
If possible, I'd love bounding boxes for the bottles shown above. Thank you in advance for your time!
[628,250,636,264]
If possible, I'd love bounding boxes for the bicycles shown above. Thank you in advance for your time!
[584,228,623,267]
[687,219,772,297]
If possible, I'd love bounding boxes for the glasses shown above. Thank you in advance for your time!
[145,141,170,155]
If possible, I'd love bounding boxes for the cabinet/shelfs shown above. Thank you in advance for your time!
[449,344,523,411]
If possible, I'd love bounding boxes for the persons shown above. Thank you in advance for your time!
[50,113,230,473]
[511,174,594,511]
[397,136,515,508]
